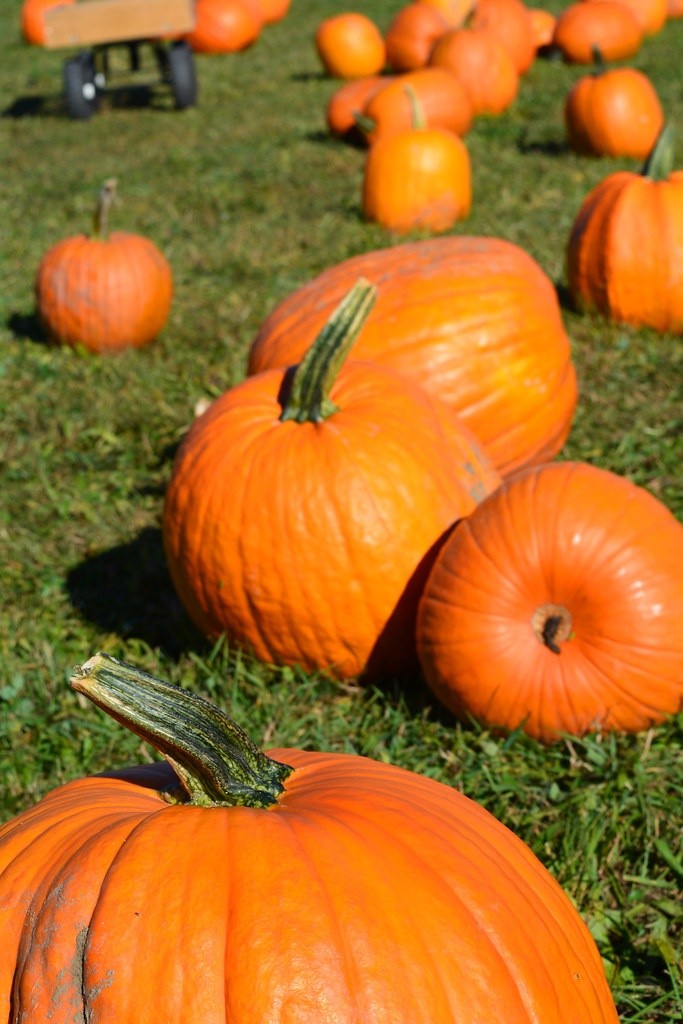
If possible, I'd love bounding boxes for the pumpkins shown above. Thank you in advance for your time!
[0,652,622,1024]
[22,0,683,750]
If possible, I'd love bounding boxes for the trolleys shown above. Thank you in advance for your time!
[42,0,198,121]
